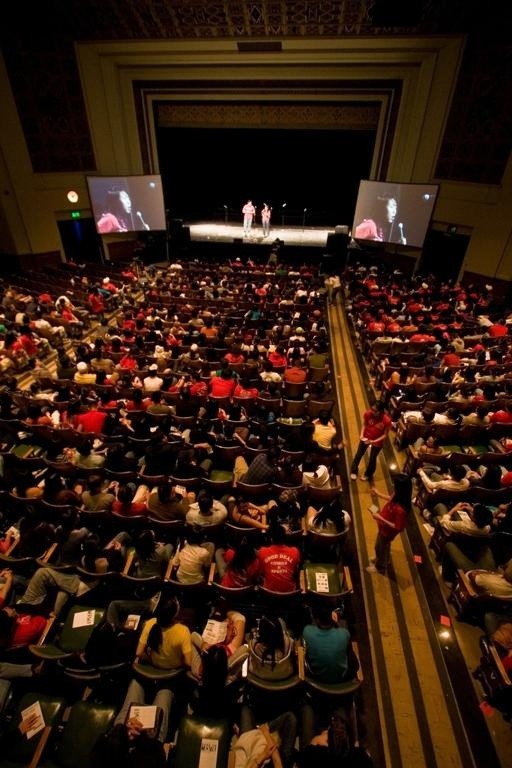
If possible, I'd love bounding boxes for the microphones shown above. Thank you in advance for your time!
[136,211,146,228]
[398,222,404,242]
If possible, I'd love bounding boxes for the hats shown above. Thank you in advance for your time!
[103,277,110,284]
[278,489,299,505]
[296,327,304,334]
[77,362,88,373]
[191,344,198,351]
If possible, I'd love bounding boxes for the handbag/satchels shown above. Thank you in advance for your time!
[223,618,234,645]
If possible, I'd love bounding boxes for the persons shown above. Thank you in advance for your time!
[97,187,150,233]
[261,205,271,238]
[242,200,256,236]
[1,259,511,768]
[356,193,407,245]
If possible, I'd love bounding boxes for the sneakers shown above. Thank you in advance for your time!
[75,579,100,598]
[366,557,390,573]
[422,508,434,525]
[150,590,162,613]
[351,473,370,481]
